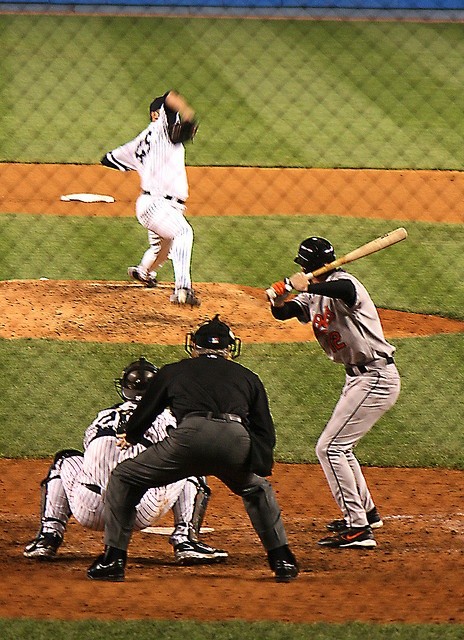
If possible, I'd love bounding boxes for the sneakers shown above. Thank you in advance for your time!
[86,555,124,582]
[174,288,200,306]
[326,509,383,531]
[23,540,57,561]
[173,542,229,565]
[128,266,157,288]
[274,560,298,583]
[317,527,377,547]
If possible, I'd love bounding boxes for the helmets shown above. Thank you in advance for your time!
[294,237,334,270]
[114,358,159,403]
[184,322,241,360]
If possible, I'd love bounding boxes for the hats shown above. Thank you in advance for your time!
[150,97,164,114]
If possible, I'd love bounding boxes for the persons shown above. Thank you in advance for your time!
[99,91,201,306]
[21,358,231,566]
[84,313,303,584]
[267,236,404,549]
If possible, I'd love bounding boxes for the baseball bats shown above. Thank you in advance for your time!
[265,227,407,298]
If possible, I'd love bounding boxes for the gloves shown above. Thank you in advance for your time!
[270,281,288,307]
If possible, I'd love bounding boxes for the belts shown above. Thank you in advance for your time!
[177,412,242,425]
[85,484,101,495]
[344,357,394,376]
[143,192,185,204]
